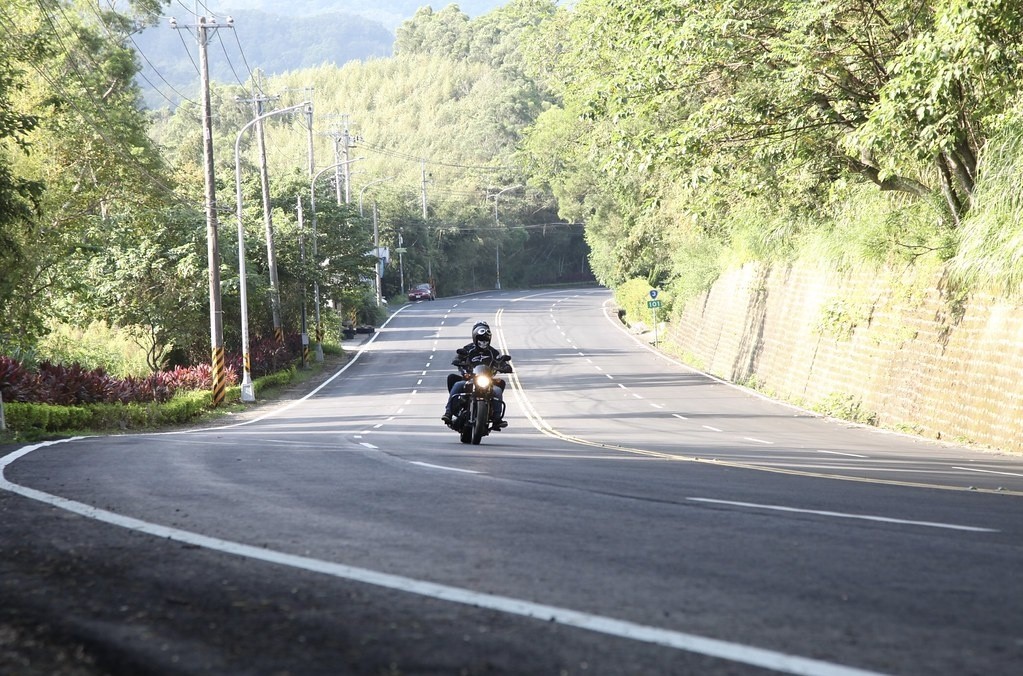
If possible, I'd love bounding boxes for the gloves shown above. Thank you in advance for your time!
[452,359,461,366]
[503,365,512,373]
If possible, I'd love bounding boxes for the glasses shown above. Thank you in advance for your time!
[478,328,492,335]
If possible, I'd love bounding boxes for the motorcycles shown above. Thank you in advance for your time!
[444,349,514,445]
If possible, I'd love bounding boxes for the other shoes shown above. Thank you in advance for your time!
[492,418,508,429]
[442,413,452,422]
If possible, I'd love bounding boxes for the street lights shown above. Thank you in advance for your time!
[358,175,397,219]
[234,100,313,402]
[310,156,366,362]
[494,184,523,290]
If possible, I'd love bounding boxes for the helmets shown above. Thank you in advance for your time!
[473,326,491,346]
[473,320,489,331]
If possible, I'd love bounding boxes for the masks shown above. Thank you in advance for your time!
[477,340,490,349]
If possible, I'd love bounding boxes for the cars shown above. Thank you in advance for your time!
[408,283,437,301]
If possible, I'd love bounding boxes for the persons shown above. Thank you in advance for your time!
[441,320,512,431]
